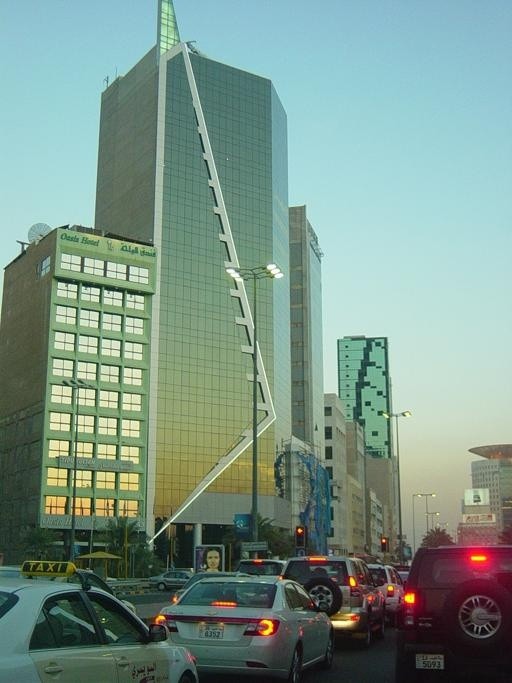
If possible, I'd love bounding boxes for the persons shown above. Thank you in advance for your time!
[202,547,222,573]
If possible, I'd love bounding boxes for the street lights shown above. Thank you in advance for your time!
[219,258,285,560]
[415,491,436,547]
[60,374,98,563]
[424,510,462,542]
[383,410,412,561]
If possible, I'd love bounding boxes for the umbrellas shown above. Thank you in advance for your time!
[74,552,121,559]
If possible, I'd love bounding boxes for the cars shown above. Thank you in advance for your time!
[0,558,199,683]
[156,575,336,681]
[147,570,194,590]
[0,564,136,618]
[172,570,253,605]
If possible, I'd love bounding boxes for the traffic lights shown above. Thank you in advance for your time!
[295,526,306,548]
[381,537,387,552]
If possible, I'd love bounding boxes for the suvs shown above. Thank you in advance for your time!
[395,563,411,583]
[394,543,512,683]
[235,558,286,580]
[277,555,388,649]
[365,562,403,624]
